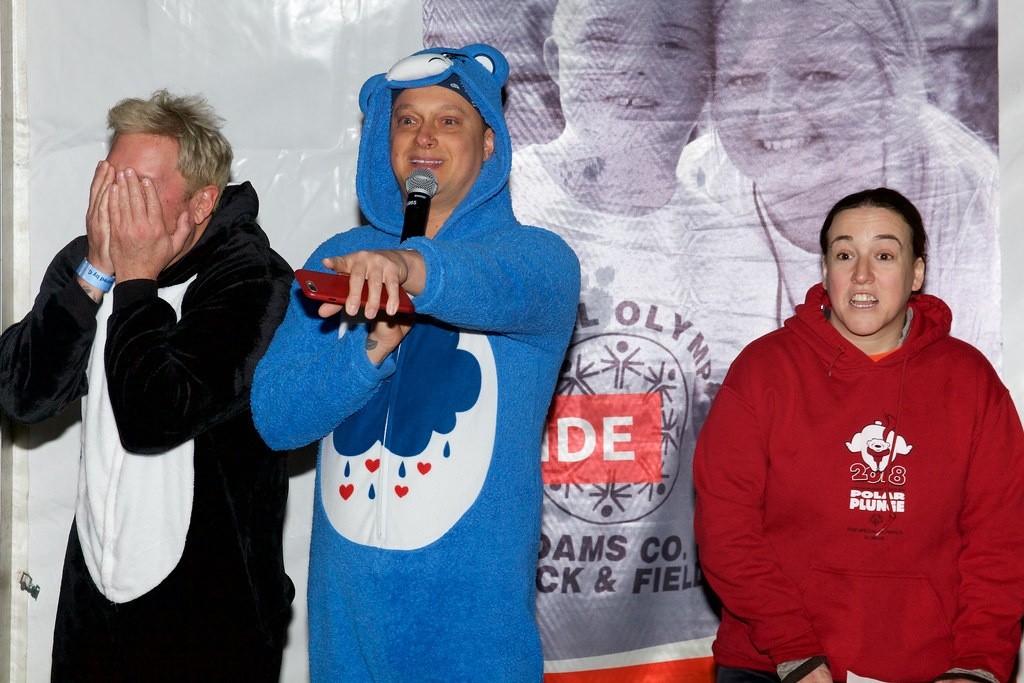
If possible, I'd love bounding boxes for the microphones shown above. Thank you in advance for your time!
[400,168,438,242]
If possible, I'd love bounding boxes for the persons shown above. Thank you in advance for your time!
[678,1,1001,379]
[691,188,1024,683]
[250,42,581,683]
[1,83,296,683]
[507,0,792,666]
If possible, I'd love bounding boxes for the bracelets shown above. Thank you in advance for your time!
[75,257,115,293]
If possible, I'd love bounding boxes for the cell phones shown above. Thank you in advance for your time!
[294,269,415,314]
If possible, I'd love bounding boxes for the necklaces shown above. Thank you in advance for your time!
[752,182,799,316]
